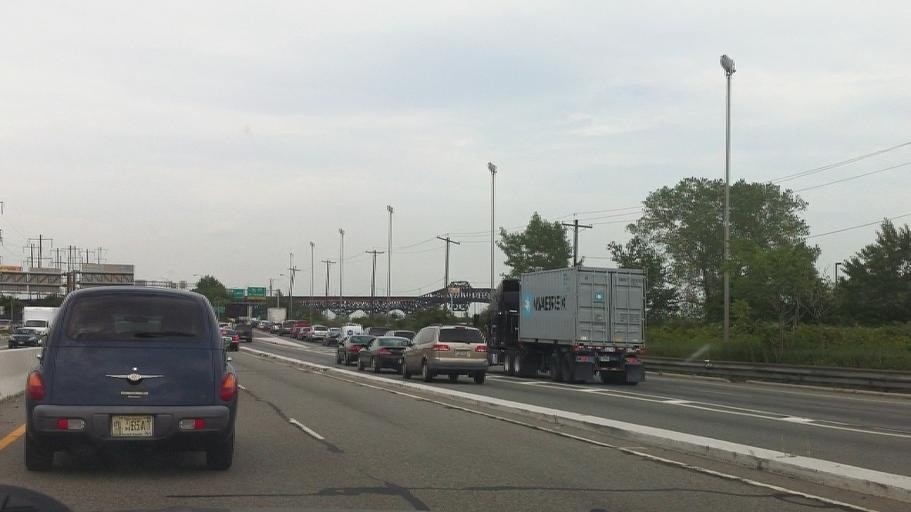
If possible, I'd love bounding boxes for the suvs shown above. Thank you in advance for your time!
[399,324,490,386]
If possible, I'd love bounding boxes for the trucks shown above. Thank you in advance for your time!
[22,305,59,340]
[490,268,649,388]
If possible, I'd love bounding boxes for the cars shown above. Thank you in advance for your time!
[357,335,414,374]
[0,319,24,333]
[8,328,48,348]
[22,285,242,474]
[335,334,374,368]
[218,316,416,353]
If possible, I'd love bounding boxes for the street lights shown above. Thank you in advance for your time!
[386,204,396,327]
[717,50,740,345]
[308,241,317,322]
[191,273,204,278]
[276,272,295,301]
[832,261,841,290]
[488,159,501,307]
[289,249,295,320]
[338,228,347,319]
